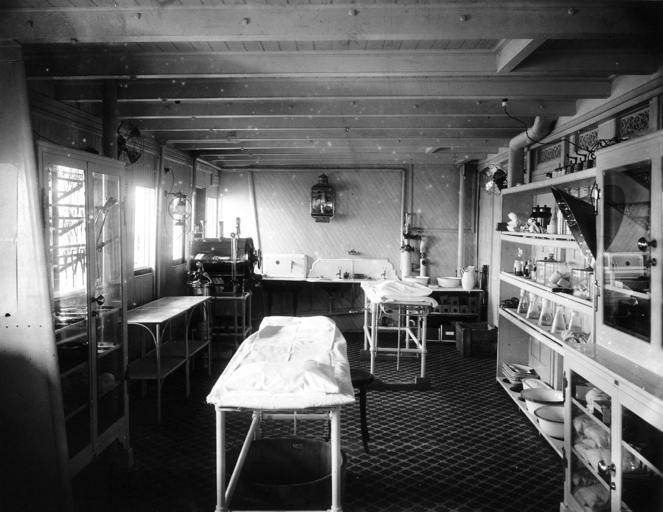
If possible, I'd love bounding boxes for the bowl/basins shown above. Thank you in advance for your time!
[436,276,461,289]
[404,276,430,288]
[520,386,563,413]
[534,406,565,441]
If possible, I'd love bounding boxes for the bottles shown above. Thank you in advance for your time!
[512,253,533,279]
[516,288,583,341]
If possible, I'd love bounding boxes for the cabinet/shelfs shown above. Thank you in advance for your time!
[35,139,135,494]
[495,129,663,512]
[426,286,485,343]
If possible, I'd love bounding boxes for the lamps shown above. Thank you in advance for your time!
[165,190,192,225]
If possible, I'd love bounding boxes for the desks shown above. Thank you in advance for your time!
[204,314,356,512]
[360,281,432,379]
[127,296,212,427]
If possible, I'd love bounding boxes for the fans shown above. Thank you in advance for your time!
[479,164,507,197]
[117,120,144,166]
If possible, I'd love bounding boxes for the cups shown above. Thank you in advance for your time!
[546,225,557,234]
